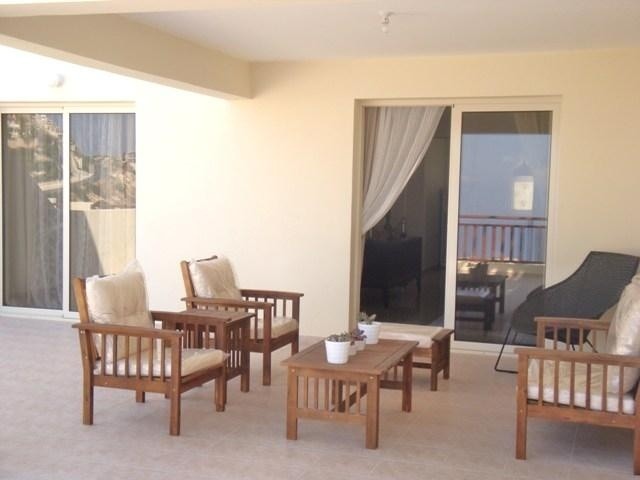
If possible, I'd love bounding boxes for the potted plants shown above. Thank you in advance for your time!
[324,312,382,365]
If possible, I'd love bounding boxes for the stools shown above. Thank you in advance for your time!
[357,320,455,391]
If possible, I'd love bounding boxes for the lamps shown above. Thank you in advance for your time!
[378,11,395,33]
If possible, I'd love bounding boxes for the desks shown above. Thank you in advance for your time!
[365,235,422,309]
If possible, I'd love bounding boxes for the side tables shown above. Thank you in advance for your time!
[162,308,256,405]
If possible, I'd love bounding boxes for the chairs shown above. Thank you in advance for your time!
[72,271,231,436]
[180,256,304,386]
[493,250,640,374]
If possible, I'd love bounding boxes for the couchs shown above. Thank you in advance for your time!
[512,271,640,475]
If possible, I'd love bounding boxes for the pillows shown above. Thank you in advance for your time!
[188,256,246,313]
[85,259,156,361]
[601,276,640,395]
